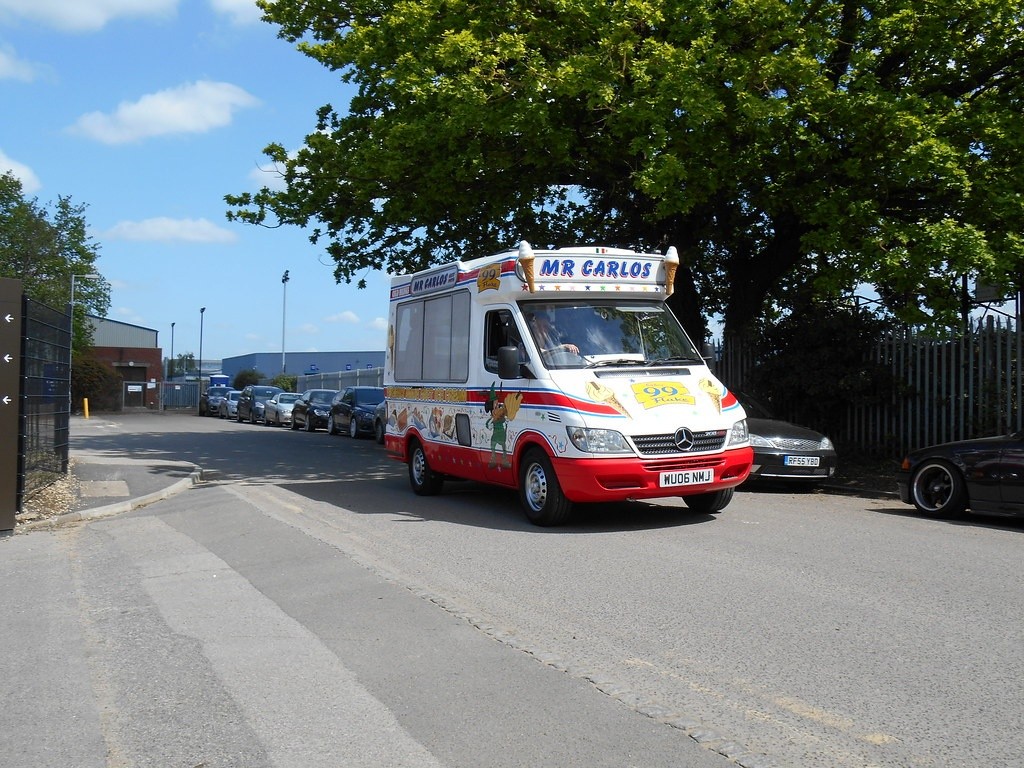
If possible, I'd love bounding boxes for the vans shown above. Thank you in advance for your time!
[383,239,755,529]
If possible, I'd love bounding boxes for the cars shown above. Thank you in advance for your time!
[896,429,1024,528]
[327,385,384,438]
[219,390,243,419]
[727,385,837,492]
[264,392,303,426]
[291,388,341,432]
[373,400,385,444]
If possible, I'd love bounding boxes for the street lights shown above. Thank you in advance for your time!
[198,307,206,407]
[281,270,290,376]
[69,272,101,419]
[171,321,175,382]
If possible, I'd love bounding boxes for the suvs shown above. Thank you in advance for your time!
[206,386,236,416]
[237,384,287,424]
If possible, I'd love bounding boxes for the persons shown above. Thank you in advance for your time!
[518,312,579,360]
